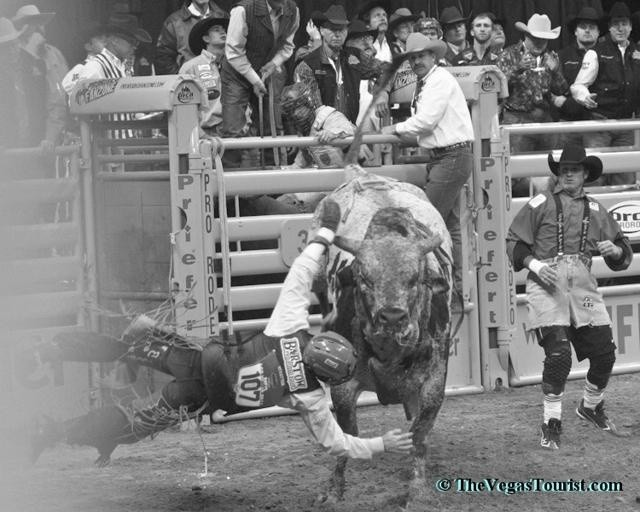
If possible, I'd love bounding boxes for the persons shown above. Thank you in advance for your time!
[0,2,640,325]
[503,143,635,450]
[16,199,415,461]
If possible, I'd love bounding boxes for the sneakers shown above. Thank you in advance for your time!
[576,398,615,431]
[540,418,562,449]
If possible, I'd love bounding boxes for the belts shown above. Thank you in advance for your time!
[430,142,470,157]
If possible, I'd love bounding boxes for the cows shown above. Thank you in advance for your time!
[310,53,454,512]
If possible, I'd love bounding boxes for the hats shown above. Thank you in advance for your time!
[549,145,602,182]
[0,5,56,44]
[107,13,153,44]
[311,5,379,44]
[567,3,632,36]
[189,17,229,56]
[515,14,561,40]
[389,8,472,63]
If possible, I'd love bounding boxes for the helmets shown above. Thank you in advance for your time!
[302,331,359,386]
[279,83,315,133]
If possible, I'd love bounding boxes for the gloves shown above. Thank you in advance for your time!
[322,200,340,230]
